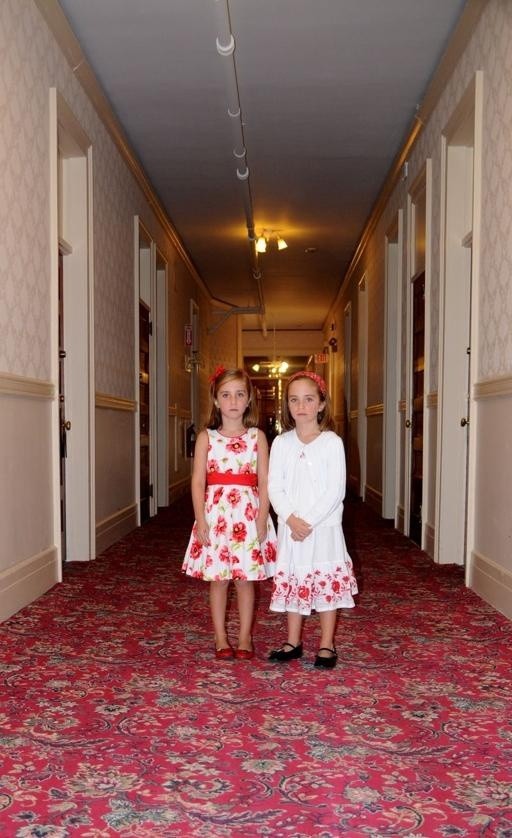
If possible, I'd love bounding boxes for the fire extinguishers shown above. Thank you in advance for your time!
[186,423,196,457]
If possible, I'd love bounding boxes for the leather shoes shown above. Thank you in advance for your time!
[314,644,337,670]
[214,639,235,660]
[267,641,304,662]
[235,642,254,660]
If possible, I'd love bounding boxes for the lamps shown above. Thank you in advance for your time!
[255,228,289,254]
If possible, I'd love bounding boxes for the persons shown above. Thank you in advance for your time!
[266,371,360,670]
[182,368,277,659]
